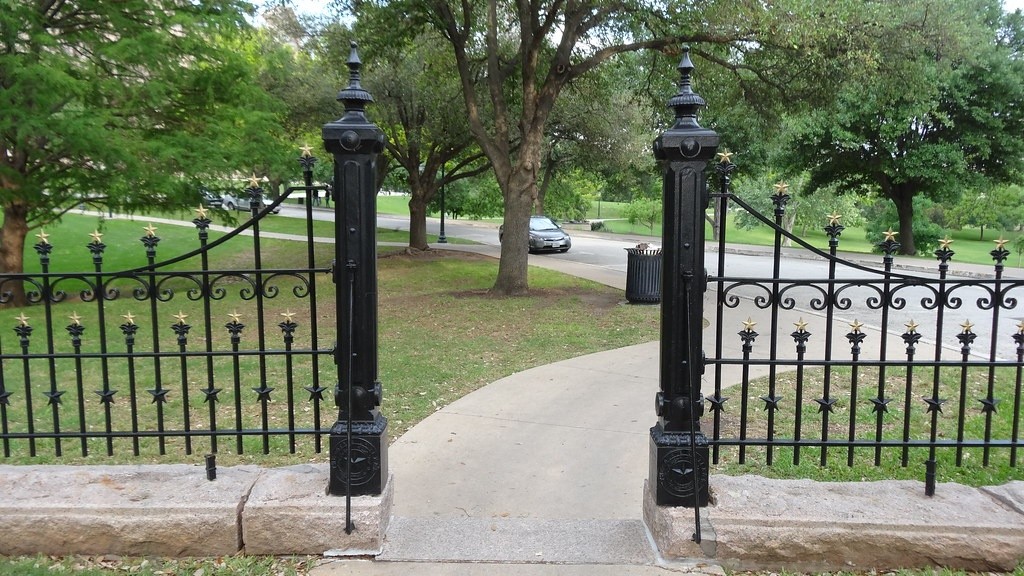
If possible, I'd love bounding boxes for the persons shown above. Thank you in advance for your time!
[79,197,85,215]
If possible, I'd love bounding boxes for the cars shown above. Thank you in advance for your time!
[224,192,281,215]
[200,190,224,209]
[499,215,571,254]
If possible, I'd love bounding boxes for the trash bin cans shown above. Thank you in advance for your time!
[625,246,661,305]
